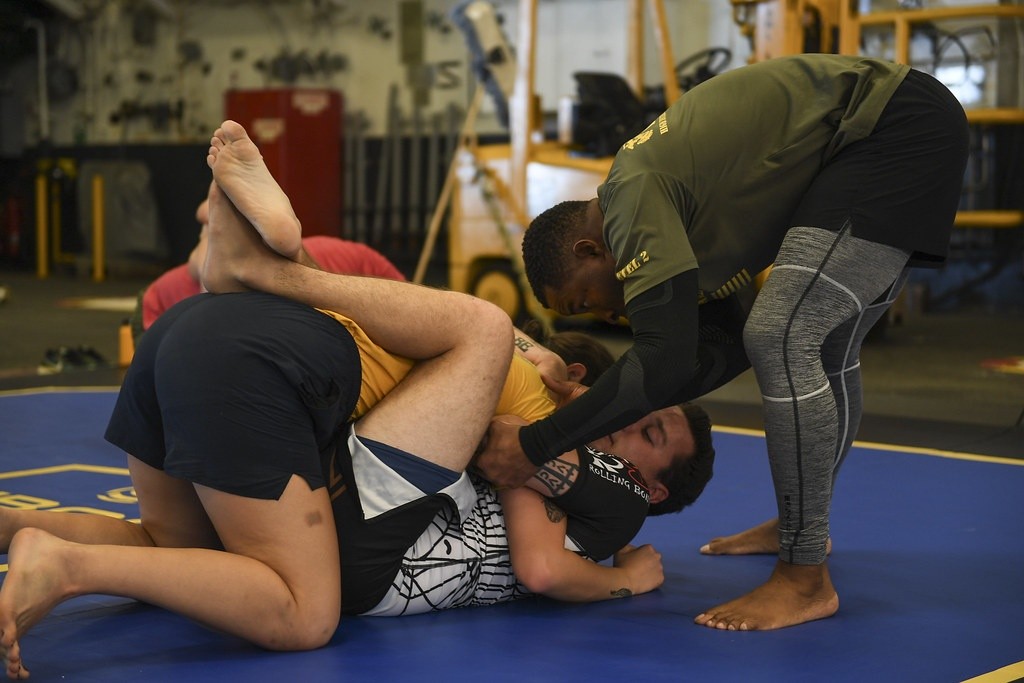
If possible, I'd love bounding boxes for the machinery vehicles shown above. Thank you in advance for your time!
[440,0,1023,340]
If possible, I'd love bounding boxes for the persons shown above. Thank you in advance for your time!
[474,51,972,633]
[0,119,716,683]
